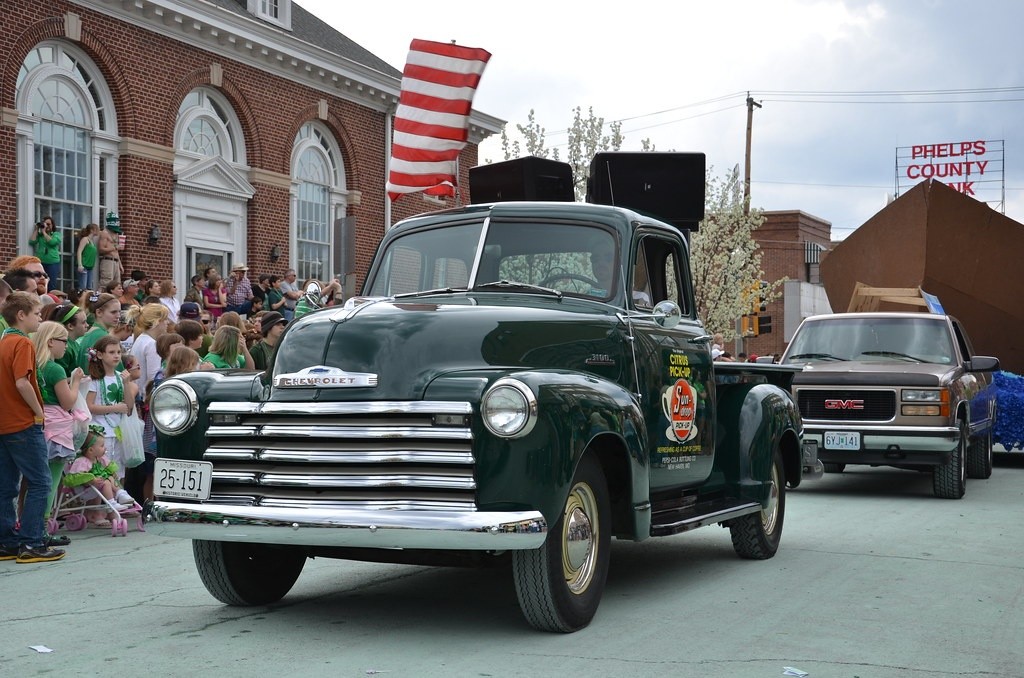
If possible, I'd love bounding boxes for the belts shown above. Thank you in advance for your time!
[102,257,118,262]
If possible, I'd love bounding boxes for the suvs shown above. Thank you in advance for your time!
[777,312,1001,500]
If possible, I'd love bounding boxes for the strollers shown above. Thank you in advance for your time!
[44,459,145,536]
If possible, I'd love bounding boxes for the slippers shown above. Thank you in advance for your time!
[87,520,112,529]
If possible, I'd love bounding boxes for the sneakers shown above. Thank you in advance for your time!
[0,542,21,560]
[118,494,134,504]
[16,544,65,563]
[107,502,128,514]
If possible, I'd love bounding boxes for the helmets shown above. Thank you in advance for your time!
[307,281,321,293]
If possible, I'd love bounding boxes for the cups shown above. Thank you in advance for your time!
[119,235,126,250]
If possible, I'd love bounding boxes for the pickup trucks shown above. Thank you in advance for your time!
[148,154,802,632]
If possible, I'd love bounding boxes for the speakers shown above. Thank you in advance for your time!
[469,156,576,205]
[587,152,706,233]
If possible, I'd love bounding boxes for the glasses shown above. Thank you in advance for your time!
[590,253,612,263]
[172,286,176,288]
[201,319,211,324]
[34,272,48,279]
[52,338,68,345]
[290,275,296,277]
[128,363,140,371]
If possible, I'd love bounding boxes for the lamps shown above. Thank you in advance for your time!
[270,242,281,263]
[148,223,160,248]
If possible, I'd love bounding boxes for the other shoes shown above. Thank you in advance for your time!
[43,535,71,546]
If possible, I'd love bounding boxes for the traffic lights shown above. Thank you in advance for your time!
[742,280,772,339]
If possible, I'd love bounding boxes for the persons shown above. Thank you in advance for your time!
[583,245,650,306]
[0,212,342,562]
[712,333,781,364]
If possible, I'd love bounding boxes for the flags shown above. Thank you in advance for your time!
[386,38,492,205]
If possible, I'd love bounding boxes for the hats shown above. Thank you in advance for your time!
[50,290,68,296]
[231,263,249,271]
[123,279,140,289]
[134,272,151,281]
[261,311,289,335]
[179,302,201,323]
[712,349,725,360]
[750,355,757,361]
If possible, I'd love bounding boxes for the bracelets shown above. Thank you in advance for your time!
[33,416,43,422]
[335,292,343,294]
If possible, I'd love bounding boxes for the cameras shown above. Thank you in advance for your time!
[37,222,44,228]
